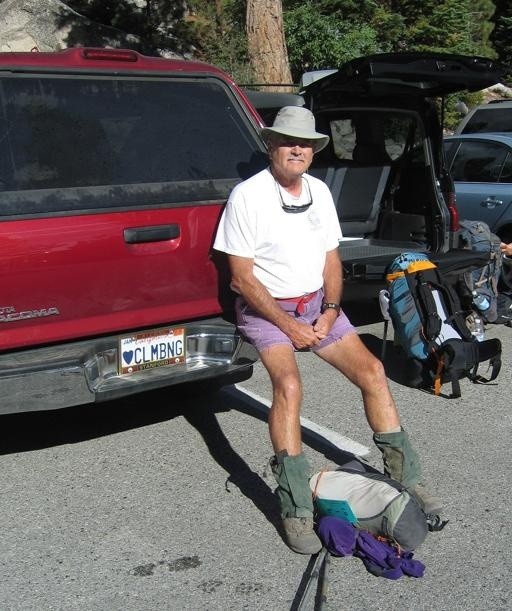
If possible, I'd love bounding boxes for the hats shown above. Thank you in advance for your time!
[260,105,330,155]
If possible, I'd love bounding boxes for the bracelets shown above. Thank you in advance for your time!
[318,302,341,317]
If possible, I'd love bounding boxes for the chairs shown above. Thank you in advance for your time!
[311,135,390,232]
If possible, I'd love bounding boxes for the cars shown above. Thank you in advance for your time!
[451,98,512,138]
[376,130,512,293]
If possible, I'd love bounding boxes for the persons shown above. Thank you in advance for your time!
[209,101,451,559]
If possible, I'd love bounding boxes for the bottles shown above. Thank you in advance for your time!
[472,290,498,323]
[470,319,483,342]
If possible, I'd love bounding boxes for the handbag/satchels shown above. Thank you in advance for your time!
[309,458,428,550]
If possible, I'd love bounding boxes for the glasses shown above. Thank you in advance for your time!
[276,177,313,214]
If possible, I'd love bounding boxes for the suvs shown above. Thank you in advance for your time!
[0,47,272,425]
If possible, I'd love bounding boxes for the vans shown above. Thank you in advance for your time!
[234,47,510,305]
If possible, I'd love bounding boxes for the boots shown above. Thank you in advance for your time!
[372,425,444,515]
[268,449,323,555]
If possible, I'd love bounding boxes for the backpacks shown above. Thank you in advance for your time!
[380,251,502,398]
[457,218,512,328]
